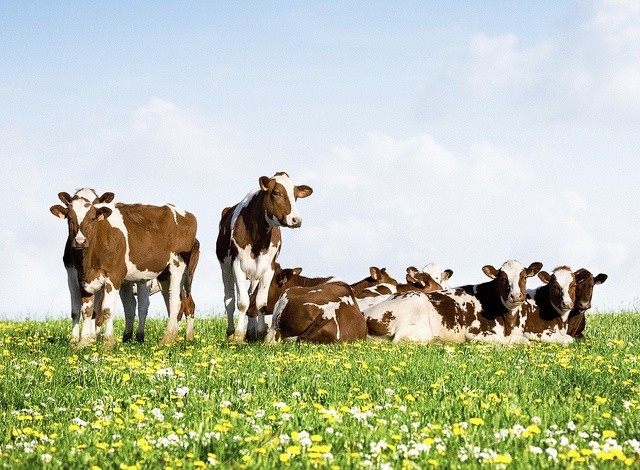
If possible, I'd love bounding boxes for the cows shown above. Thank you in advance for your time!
[355,272,443,313]
[348,267,398,293]
[50,202,200,351]
[362,260,543,345]
[247,263,368,344]
[567,268,608,337]
[517,265,590,345]
[216,172,313,343]
[58,188,150,342]
[406,264,453,289]
